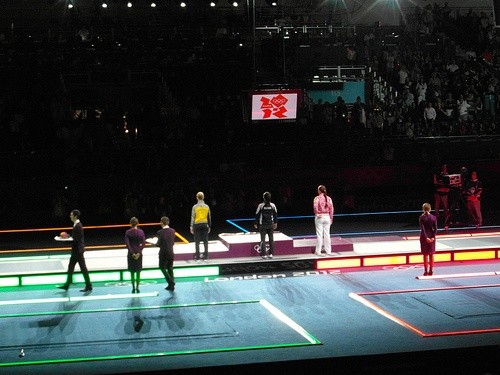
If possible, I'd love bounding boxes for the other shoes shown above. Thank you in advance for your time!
[428,270,433,275]
[262,256,267,259]
[136,288,140,293]
[79,287,92,292]
[423,271,428,276]
[165,282,175,291]
[57,285,68,290]
[132,288,135,293]
[268,254,272,258]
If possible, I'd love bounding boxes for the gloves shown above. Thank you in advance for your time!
[208,228,210,233]
[190,226,194,234]
[272,222,278,230]
[254,224,258,229]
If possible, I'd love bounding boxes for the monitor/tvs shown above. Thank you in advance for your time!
[248,89,303,123]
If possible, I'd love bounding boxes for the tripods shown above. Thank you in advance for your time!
[444,194,478,225]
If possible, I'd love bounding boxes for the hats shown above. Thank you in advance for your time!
[263,192,271,198]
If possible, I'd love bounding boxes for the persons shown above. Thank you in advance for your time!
[125,217,145,293]
[154,217,176,290]
[419,203,437,276]
[56,210,93,292]
[190,192,211,260]
[0,0,500,226]
[313,185,334,255]
[255,192,278,259]
